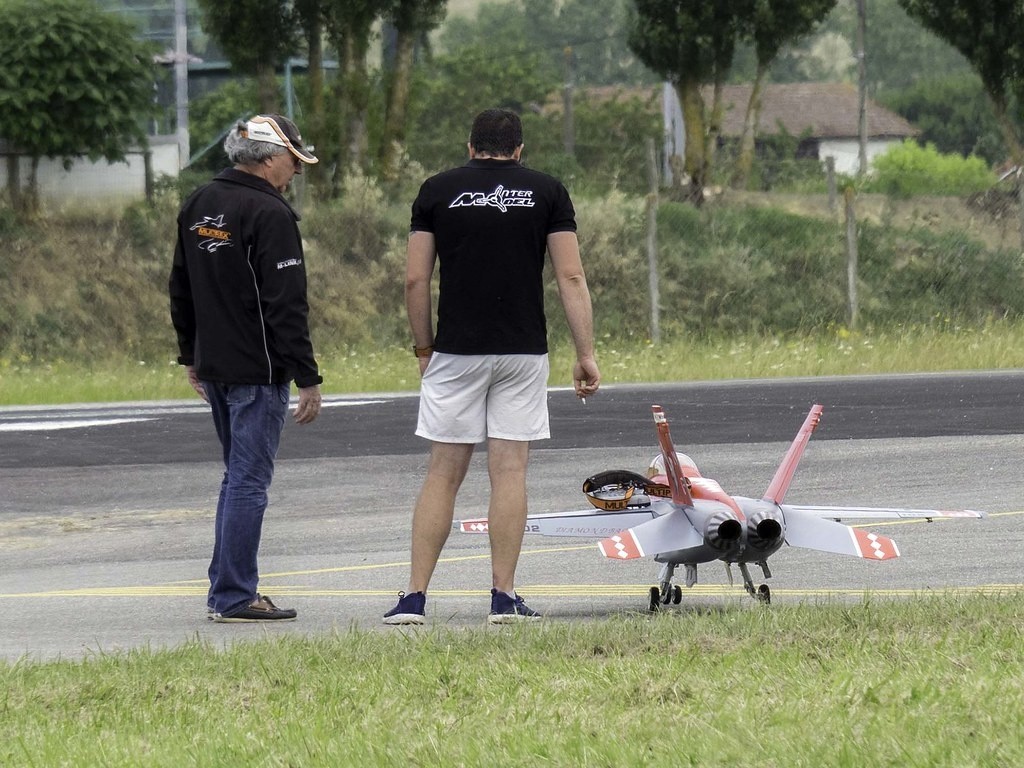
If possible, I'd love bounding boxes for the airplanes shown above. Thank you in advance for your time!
[449,404,985,613]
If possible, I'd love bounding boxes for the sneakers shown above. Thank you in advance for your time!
[489,588,543,624]
[382,590,426,623]
[206,595,297,623]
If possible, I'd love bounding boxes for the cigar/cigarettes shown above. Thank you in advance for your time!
[581,398,586,404]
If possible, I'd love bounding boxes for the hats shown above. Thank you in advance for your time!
[237,114,319,164]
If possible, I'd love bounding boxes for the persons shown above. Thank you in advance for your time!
[168,112,323,622]
[383,109,600,623]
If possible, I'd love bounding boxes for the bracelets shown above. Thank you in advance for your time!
[413,345,434,358]
